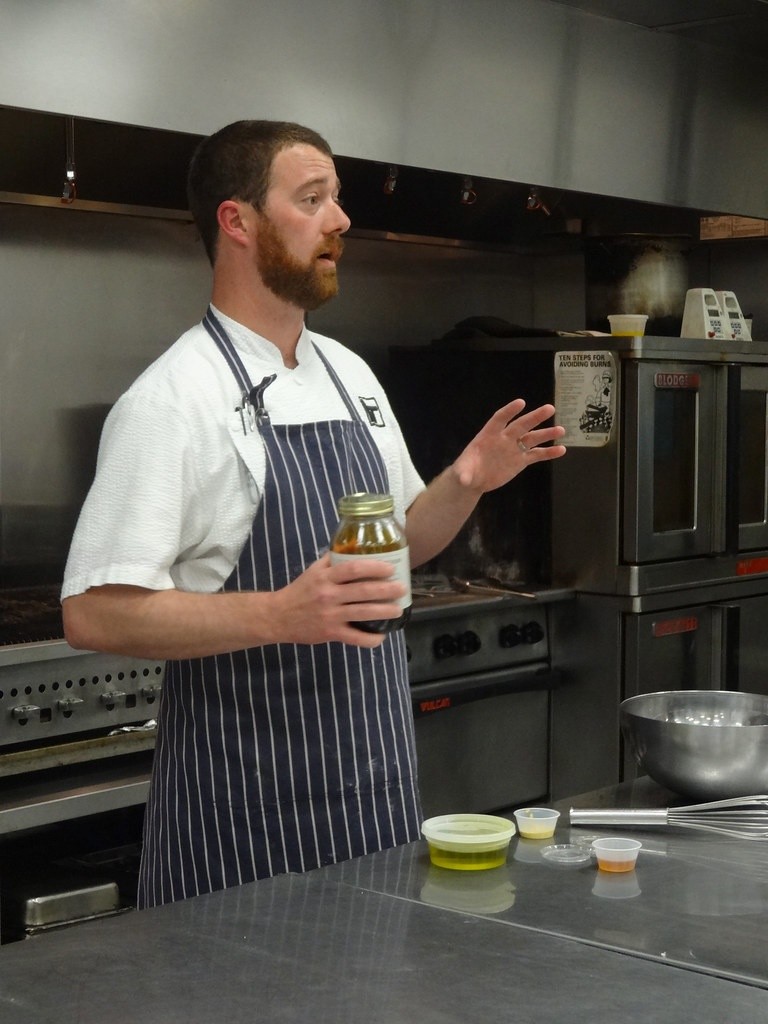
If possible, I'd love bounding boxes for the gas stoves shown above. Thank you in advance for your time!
[0,564,574,754]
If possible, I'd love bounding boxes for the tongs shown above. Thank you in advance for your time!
[452,576,538,601]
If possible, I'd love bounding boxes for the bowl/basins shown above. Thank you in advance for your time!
[618,690,768,799]
[607,314,650,337]
[420,813,516,871]
[592,837,643,873]
[512,807,561,840]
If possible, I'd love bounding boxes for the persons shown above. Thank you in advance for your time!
[61,118,567,919]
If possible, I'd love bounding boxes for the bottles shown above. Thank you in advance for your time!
[330,491,413,634]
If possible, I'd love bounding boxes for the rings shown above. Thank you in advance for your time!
[518,441,530,451]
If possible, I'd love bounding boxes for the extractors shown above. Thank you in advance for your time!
[2,0,768,263]
